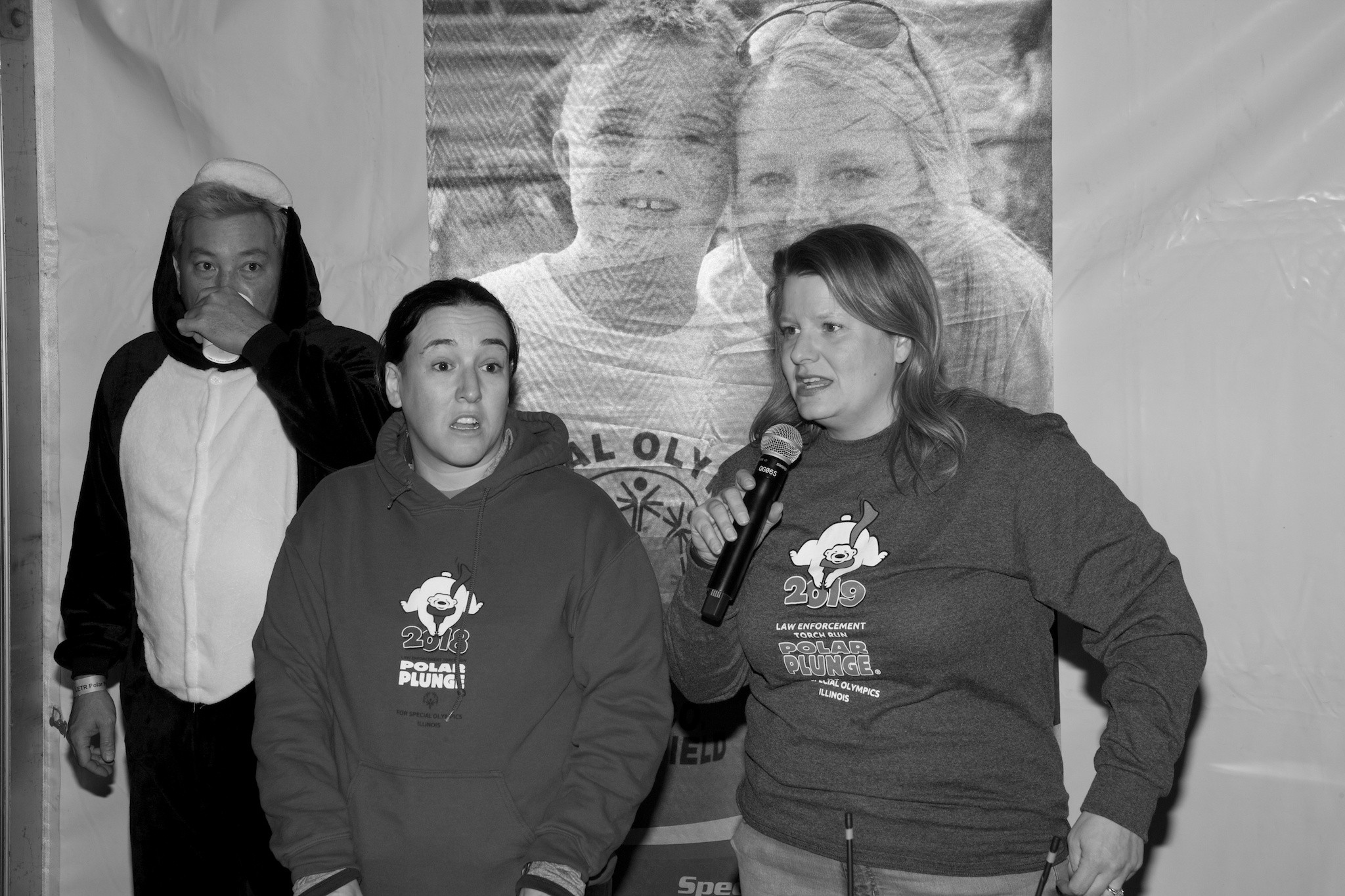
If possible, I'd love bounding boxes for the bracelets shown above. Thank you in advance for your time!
[72,675,108,698]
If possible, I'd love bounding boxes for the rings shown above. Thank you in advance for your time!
[1107,886,1124,896]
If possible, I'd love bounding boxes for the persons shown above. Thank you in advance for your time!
[467,0,1053,607]
[250,277,674,896]
[54,161,399,895]
[663,223,1208,896]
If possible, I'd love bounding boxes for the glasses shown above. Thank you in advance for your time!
[735,0,918,82]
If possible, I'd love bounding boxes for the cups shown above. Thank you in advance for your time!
[202,292,253,364]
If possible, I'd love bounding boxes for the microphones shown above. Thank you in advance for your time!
[699,423,804,623]
